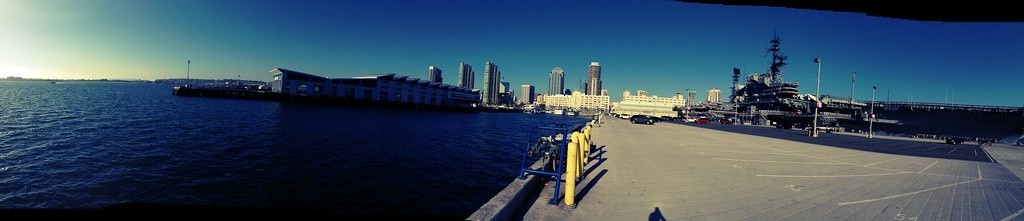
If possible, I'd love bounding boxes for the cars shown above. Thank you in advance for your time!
[695,118,711,123]
[618,113,632,120]
[650,117,662,123]
[630,114,654,125]
[661,116,674,122]
[946,137,966,145]
[721,119,733,125]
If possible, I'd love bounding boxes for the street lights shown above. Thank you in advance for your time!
[812,57,822,138]
[867,86,877,139]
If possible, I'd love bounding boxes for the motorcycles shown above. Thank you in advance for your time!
[978,139,994,147]
